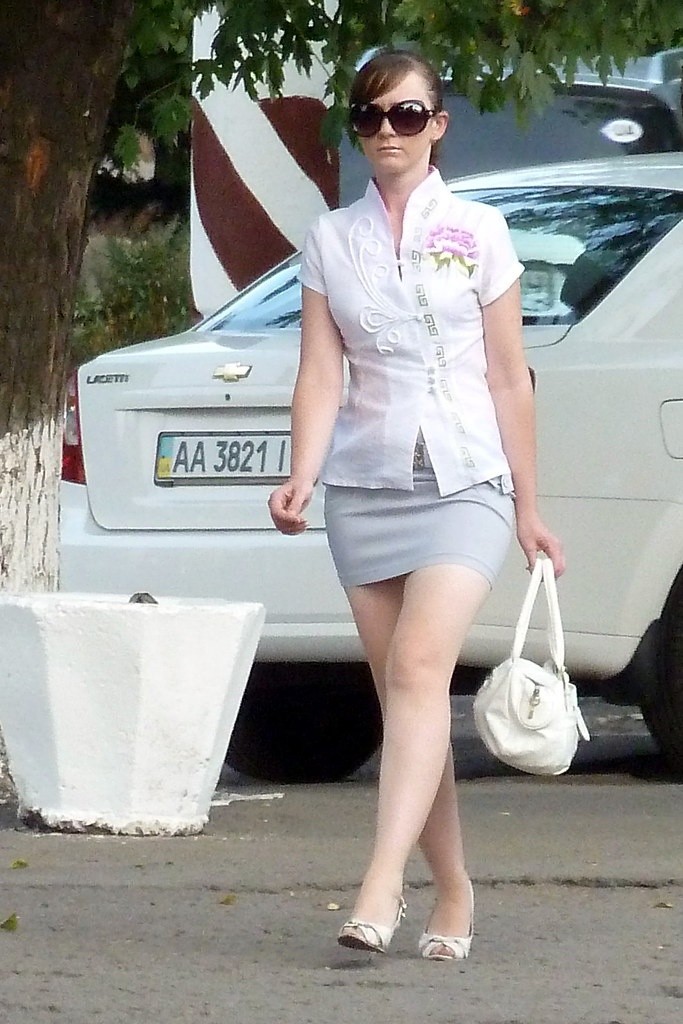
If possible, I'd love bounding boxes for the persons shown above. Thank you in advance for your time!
[267,49,565,960]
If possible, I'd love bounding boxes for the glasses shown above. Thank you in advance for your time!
[348,99,436,136]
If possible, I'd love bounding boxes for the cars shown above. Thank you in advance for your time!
[59,149,682,777]
[340,45,683,201]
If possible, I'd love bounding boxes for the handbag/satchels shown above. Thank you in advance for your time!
[472,558,589,777]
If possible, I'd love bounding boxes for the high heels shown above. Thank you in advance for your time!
[336,896,406,961]
[427,878,474,952]
